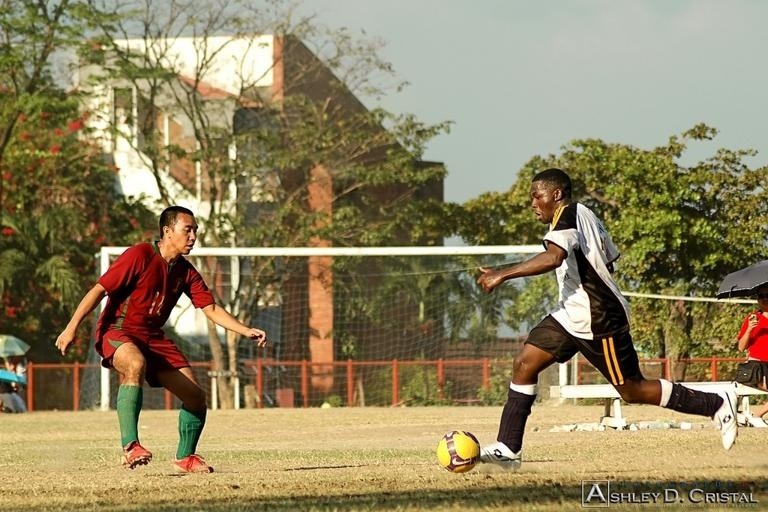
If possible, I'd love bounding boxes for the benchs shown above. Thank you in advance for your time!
[549,381,768,431]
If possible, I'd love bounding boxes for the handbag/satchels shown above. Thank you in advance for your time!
[736,363,762,386]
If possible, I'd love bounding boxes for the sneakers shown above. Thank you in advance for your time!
[480,441,522,469]
[748,414,768,427]
[711,392,737,450]
[175,454,213,474]
[123,440,152,469]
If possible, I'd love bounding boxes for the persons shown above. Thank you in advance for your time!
[478,169,739,472]
[733,292,768,433]
[54,205,268,474]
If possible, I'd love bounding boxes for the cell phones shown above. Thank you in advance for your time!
[751,313,758,322]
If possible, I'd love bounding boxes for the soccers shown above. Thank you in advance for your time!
[438,431,482,472]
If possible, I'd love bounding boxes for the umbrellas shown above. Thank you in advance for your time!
[714,258,767,298]
